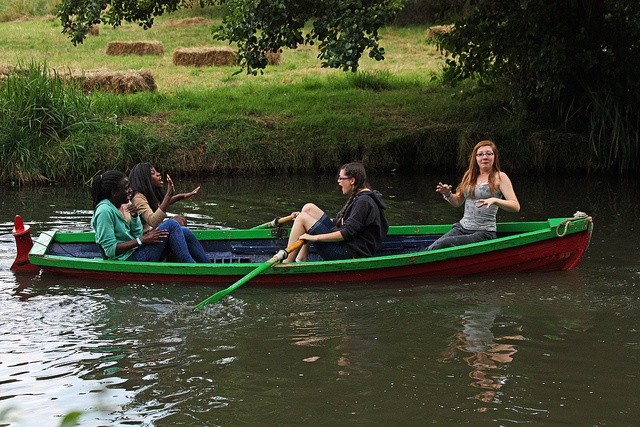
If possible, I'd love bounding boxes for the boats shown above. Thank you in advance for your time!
[29,211,594,287]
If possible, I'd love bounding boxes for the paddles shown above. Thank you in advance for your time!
[191,238,306,312]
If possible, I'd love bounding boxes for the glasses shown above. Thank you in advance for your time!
[339,176,351,181]
[476,153,494,157]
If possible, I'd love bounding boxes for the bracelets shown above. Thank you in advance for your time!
[136,237,143,246]
[443,191,452,198]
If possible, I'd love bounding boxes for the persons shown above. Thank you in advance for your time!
[282,162,389,262]
[128,162,201,234]
[90,169,212,263]
[426,141,520,250]
